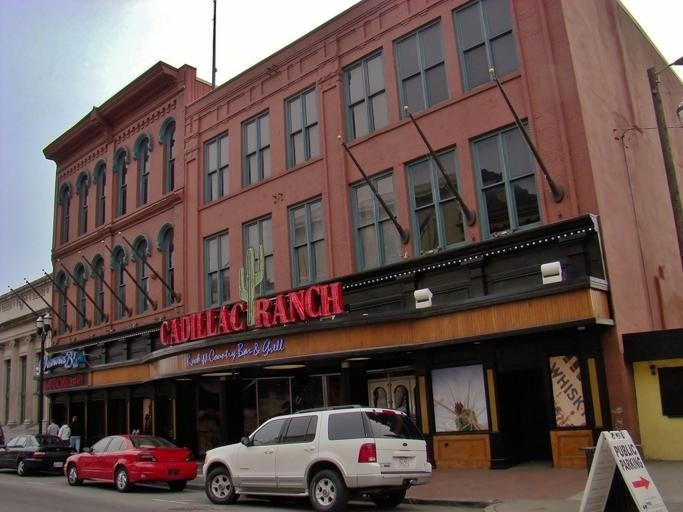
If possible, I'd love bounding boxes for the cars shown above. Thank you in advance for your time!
[63,435,198,493]
[0,434,77,477]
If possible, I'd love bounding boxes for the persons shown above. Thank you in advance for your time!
[45,418,59,436]
[68,415,82,454]
[57,424,71,447]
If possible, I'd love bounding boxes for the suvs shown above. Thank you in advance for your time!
[201,404,432,511]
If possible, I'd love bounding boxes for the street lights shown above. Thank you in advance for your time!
[36,313,53,434]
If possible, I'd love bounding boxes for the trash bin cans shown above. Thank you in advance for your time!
[578,445,643,512]
